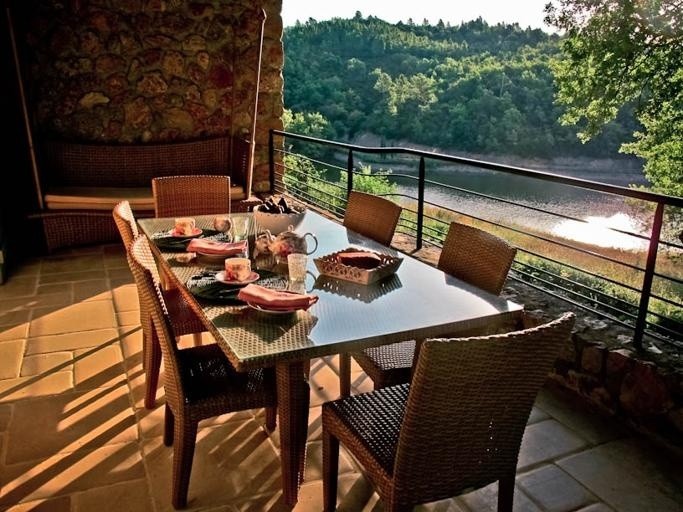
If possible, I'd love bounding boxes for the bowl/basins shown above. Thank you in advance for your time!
[253,204,306,233]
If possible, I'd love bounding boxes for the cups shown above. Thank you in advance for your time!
[175,218,195,234]
[225,258,251,281]
[287,253,308,281]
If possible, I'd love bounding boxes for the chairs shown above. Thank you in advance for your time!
[151,174,231,218]
[339,222,517,403]
[125,233,310,510]
[111,200,209,409]
[321,311,576,512]
[341,189,403,247]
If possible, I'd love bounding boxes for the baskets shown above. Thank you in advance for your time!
[313,247,403,285]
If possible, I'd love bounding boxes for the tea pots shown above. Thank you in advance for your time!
[264,225,318,264]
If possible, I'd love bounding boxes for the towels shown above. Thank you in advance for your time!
[186,237,248,255]
[237,283,319,311]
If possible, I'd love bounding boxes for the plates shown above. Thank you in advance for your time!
[246,290,301,314]
[168,228,202,236]
[216,270,260,285]
[197,251,230,257]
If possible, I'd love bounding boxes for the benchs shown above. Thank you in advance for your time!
[27,133,263,257]
